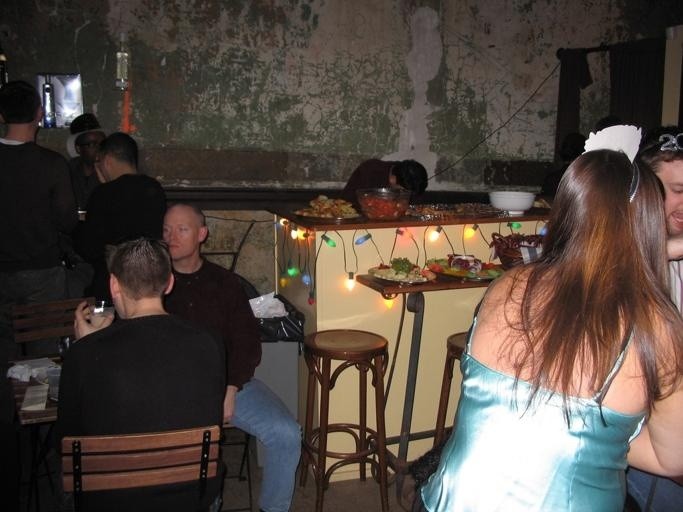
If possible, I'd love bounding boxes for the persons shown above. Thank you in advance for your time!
[626,126,683,511]
[72,134,168,299]
[39,235,224,512]
[67,115,109,208]
[418,149,680,512]
[153,206,302,510]
[2,81,77,435]
[344,158,427,201]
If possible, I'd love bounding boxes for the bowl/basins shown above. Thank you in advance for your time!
[491,191,534,215]
[359,188,412,222]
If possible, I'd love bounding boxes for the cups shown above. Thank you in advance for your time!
[47,369,61,402]
[88,300,114,328]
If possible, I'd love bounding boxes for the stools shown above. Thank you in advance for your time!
[409,331,470,510]
[299,329,390,512]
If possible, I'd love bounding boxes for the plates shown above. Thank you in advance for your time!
[428,260,502,283]
[412,203,502,221]
[368,264,434,285]
[291,209,360,223]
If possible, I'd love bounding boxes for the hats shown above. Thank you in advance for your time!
[67,113,111,159]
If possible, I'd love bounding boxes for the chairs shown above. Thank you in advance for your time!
[220,427,252,512]
[10,297,98,367]
[48,425,222,512]
